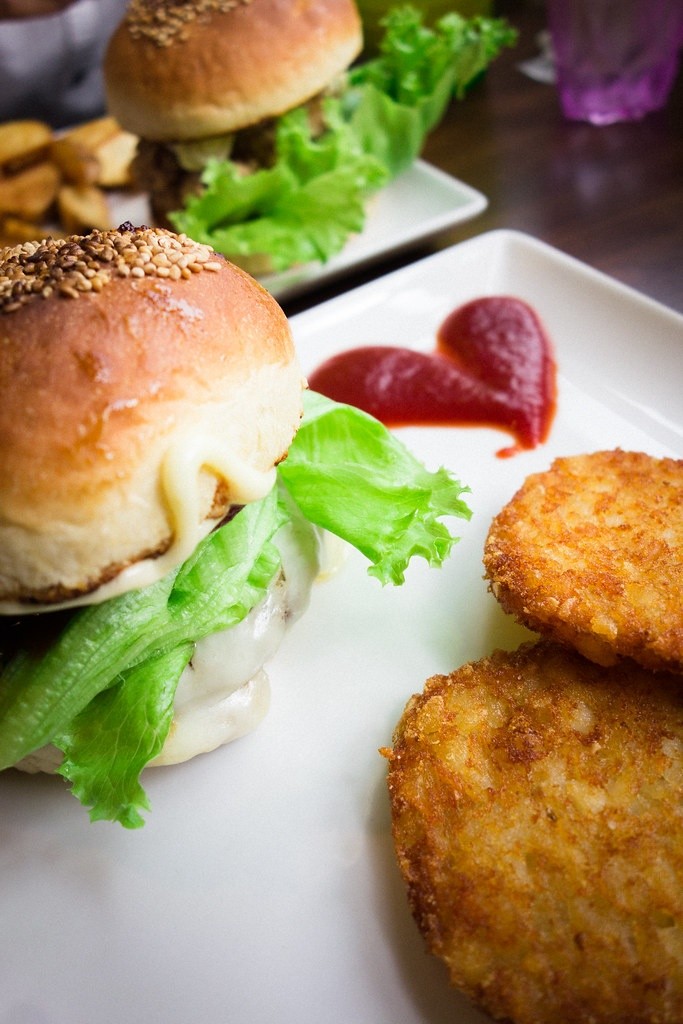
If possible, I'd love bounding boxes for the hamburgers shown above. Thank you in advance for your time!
[0,225,468,826]
[103,0,510,273]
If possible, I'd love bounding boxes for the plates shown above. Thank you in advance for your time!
[0,228,683,1024]
[39,124,488,300]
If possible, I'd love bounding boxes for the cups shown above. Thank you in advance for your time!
[547,0,683,126]
